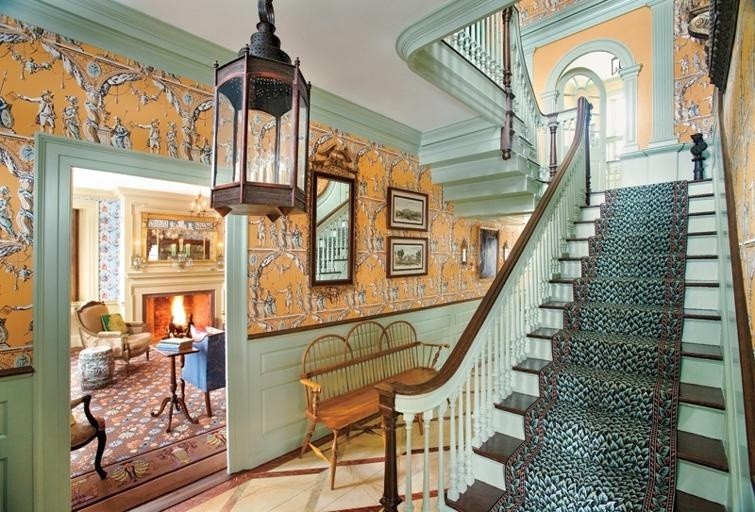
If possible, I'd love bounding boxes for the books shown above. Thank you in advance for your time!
[156,337,194,352]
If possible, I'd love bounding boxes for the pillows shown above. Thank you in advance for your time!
[101,312,128,338]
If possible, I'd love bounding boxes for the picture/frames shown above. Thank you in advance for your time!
[387,187,429,277]
[476,225,501,281]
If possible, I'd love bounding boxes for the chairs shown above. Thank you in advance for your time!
[70,394,110,480]
[180,327,226,419]
[73,300,151,378]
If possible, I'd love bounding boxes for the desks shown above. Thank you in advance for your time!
[148,341,200,433]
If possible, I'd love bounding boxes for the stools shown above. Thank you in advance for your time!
[78,345,114,390]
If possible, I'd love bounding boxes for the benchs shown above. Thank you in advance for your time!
[298,320,454,489]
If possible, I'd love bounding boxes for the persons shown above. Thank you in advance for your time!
[515,1,581,26]
[673,49,712,141]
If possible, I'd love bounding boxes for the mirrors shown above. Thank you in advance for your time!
[141,210,219,266]
[308,158,358,286]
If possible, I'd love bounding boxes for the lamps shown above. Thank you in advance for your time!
[209,1,311,221]
